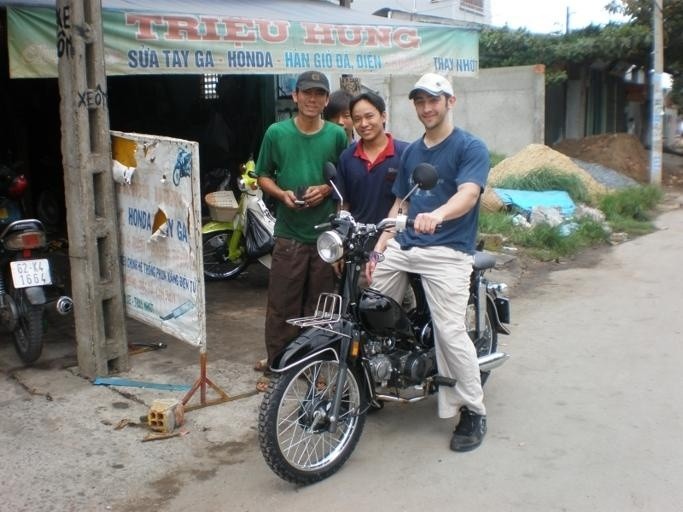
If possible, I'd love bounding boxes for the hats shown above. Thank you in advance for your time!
[296,71,330,93]
[408,73,454,98]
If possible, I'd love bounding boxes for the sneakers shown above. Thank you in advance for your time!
[450,406,488,452]
[342,395,382,415]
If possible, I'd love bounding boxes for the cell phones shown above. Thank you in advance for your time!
[294,194,306,205]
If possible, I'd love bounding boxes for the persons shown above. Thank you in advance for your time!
[322,88,355,145]
[255,69,347,371]
[366,74,491,451]
[331,92,416,279]
[627,118,635,134]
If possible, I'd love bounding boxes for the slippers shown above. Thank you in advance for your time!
[318,374,335,395]
[255,356,271,370]
[254,372,272,392]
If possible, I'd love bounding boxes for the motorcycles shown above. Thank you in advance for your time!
[201,152,276,281]
[259,161,511,486]
[0,168,73,362]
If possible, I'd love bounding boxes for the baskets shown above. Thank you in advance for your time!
[205,191,238,224]
